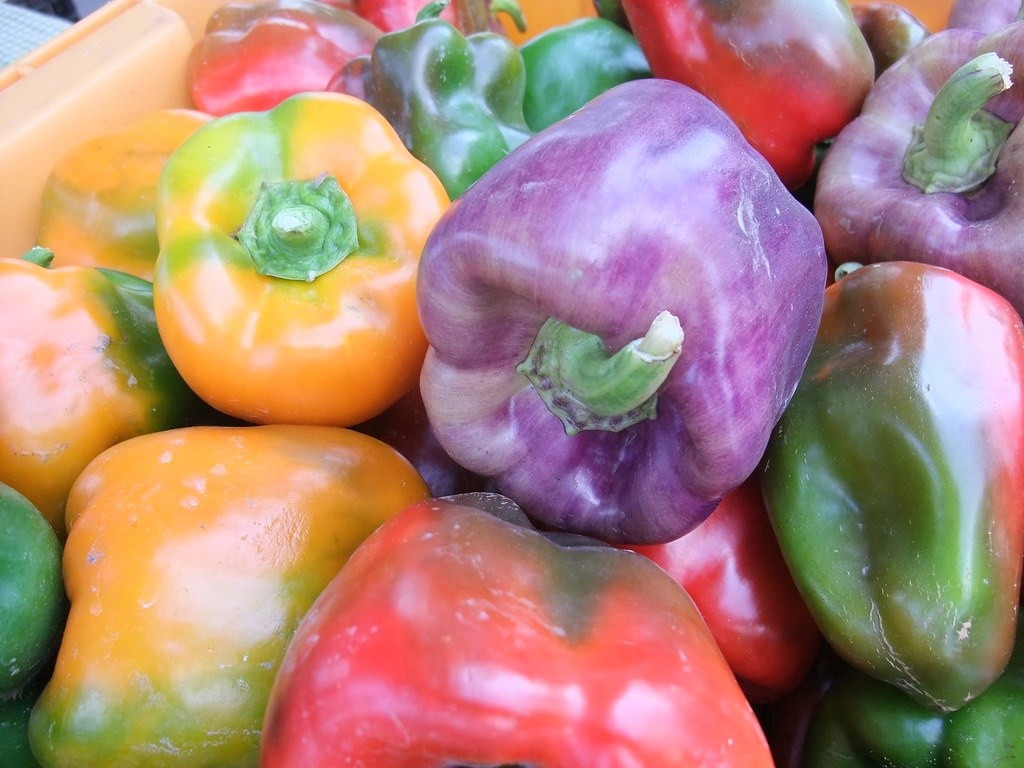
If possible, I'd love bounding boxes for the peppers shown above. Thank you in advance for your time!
[0,0,1023,768]
